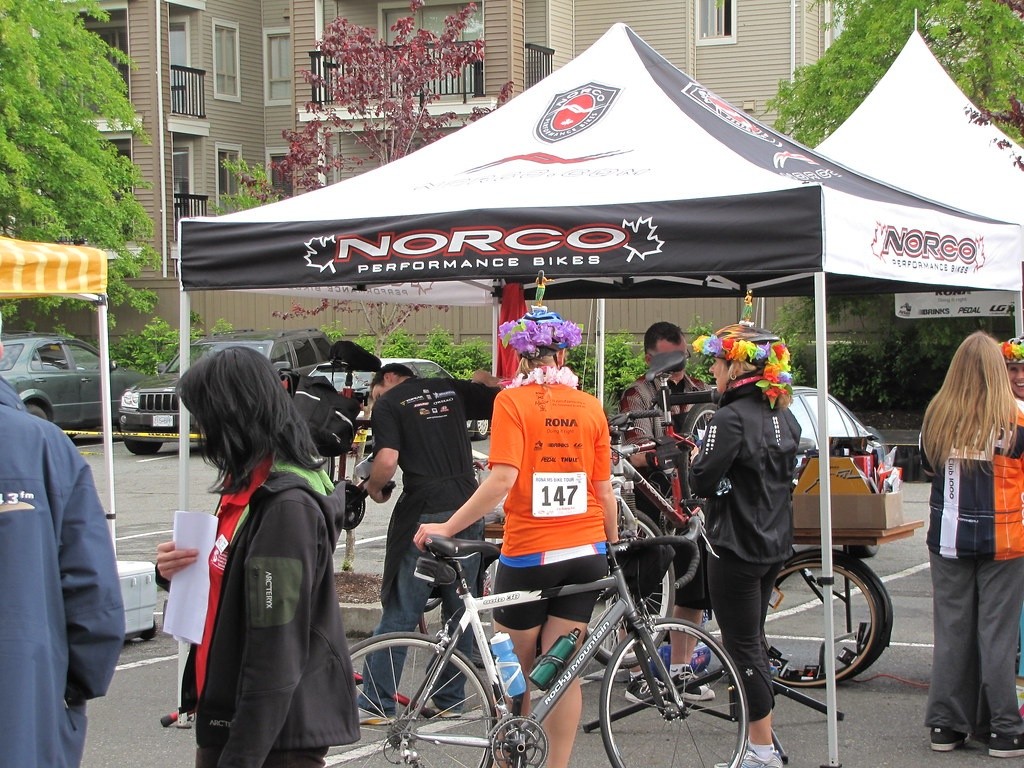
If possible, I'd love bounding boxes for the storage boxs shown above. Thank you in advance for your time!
[793,490,902,528]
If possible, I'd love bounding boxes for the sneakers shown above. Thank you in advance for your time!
[625,671,673,710]
[668,666,717,701]
[423,705,463,719]
[352,703,399,726]
[713,735,784,768]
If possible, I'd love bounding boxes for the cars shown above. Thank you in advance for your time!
[305,358,489,445]
[788,385,892,487]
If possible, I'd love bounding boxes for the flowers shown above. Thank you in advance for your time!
[696,335,793,410]
[498,320,584,354]
[999,341,1024,361]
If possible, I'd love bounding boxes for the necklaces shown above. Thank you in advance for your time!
[502,367,578,395]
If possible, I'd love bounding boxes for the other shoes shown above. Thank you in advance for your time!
[988,732,1024,758]
[930,726,968,752]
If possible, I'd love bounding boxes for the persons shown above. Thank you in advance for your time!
[154,347,361,768]
[620,320,716,705]
[358,363,516,725]
[915,332,1024,758]
[686,323,801,768]
[996,339,1024,414]
[412,306,617,768]
[1,384,126,767]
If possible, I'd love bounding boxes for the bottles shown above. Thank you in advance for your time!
[529,628,581,691]
[490,631,527,697]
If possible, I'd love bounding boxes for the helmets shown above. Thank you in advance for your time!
[1003,338,1024,364]
[513,312,570,350]
[704,324,782,366]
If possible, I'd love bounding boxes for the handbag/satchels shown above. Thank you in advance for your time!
[280,368,365,458]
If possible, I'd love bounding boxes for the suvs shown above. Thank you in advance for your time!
[0,329,151,433]
[116,329,334,455]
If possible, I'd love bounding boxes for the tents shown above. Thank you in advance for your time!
[174,24,1024,768]
[811,9,1024,325]
[0,237,117,573]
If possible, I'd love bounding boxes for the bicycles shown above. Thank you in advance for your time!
[324,352,892,768]
